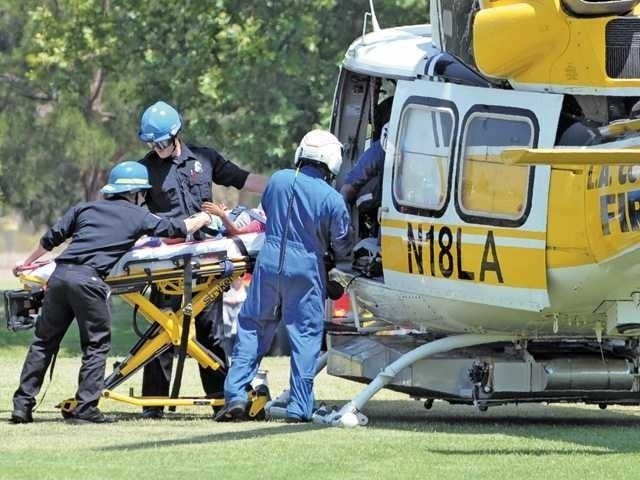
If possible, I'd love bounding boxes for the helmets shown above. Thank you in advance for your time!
[137,101,184,143]
[99,161,154,194]
[294,129,345,176]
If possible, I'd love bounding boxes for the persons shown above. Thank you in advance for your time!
[130,201,266,249]
[335,121,402,240]
[213,129,353,423]
[133,101,272,420]
[12,161,212,424]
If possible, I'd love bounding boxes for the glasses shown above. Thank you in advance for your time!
[145,140,172,150]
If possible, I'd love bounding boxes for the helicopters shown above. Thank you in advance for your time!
[265,0,640,428]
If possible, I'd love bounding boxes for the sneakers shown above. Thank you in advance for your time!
[142,407,164,418]
[213,401,246,422]
[12,409,33,422]
[62,406,119,424]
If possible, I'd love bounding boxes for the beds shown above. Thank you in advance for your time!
[4,226,272,417]
